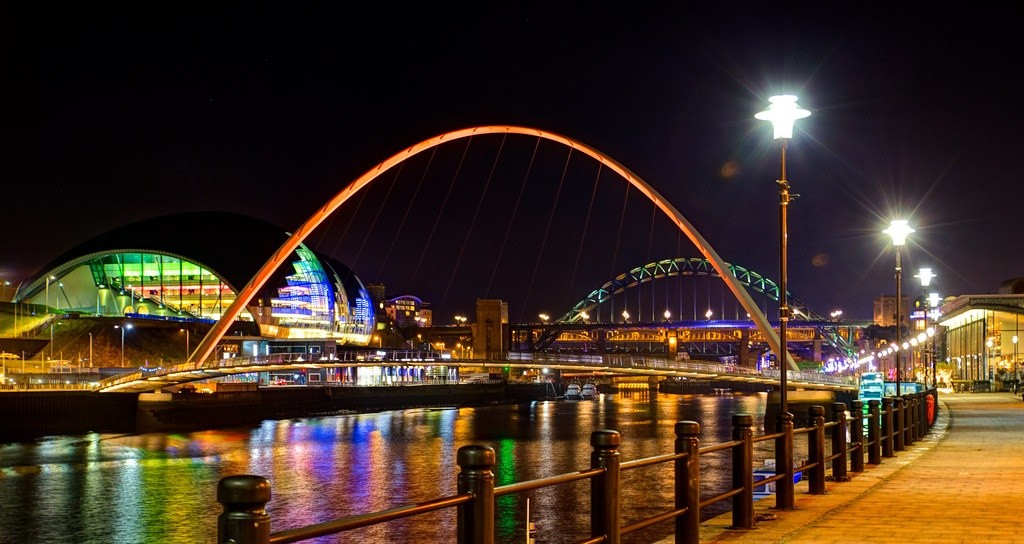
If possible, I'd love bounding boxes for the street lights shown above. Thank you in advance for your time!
[753,94,813,508]
[882,218,916,452]
[827,265,1020,420]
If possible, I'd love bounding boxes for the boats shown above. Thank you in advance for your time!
[580,380,598,400]
[565,377,582,400]
